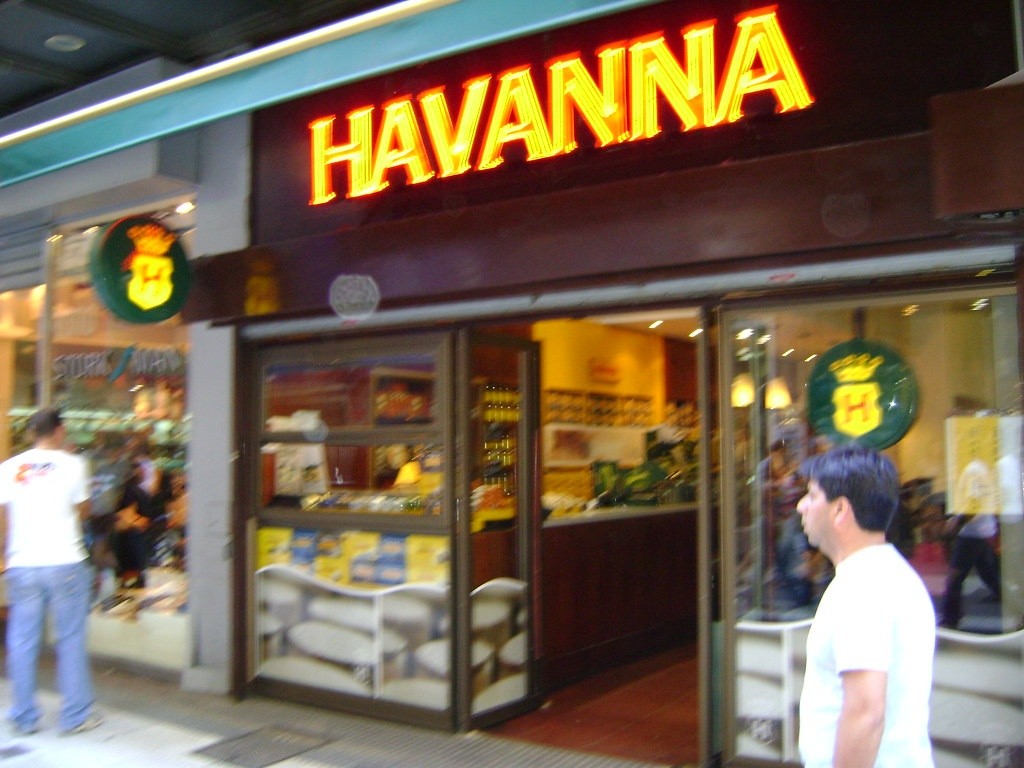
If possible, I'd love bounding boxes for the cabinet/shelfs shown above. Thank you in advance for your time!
[542,386,712,503]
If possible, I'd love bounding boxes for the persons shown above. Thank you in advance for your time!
[796,445,936,768]
[0,406,102,737]
[942,437,1001,631]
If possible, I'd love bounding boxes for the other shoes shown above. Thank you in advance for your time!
[61,711,103,736]
